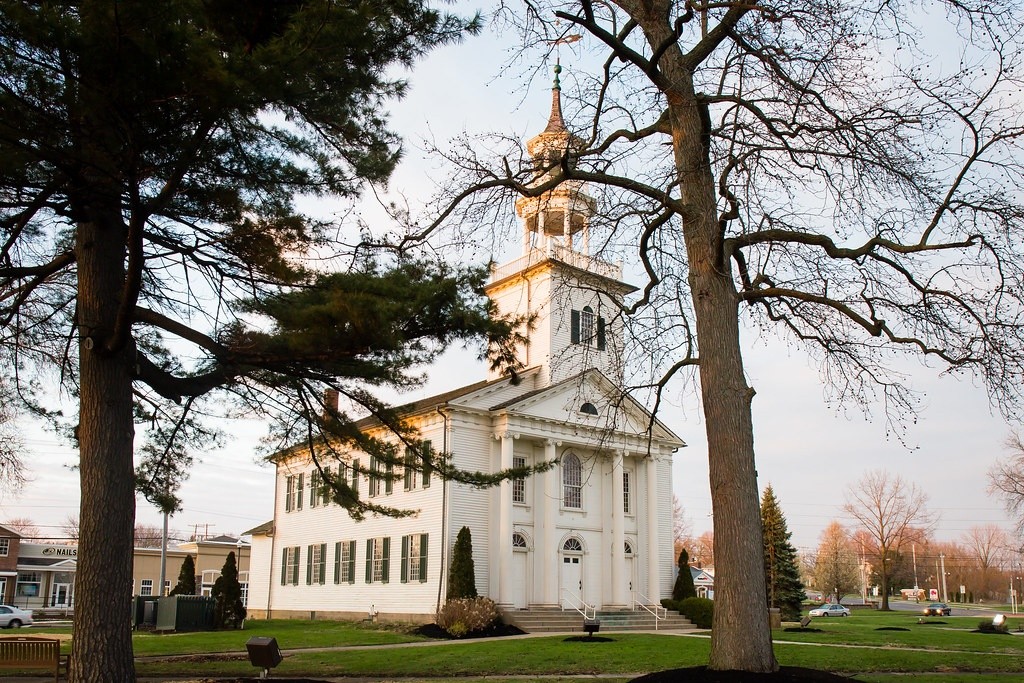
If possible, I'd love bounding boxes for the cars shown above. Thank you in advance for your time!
[810,595,822,601]
[0,605,33,629]
[808,603,851,617]
[923,603,951,616]
